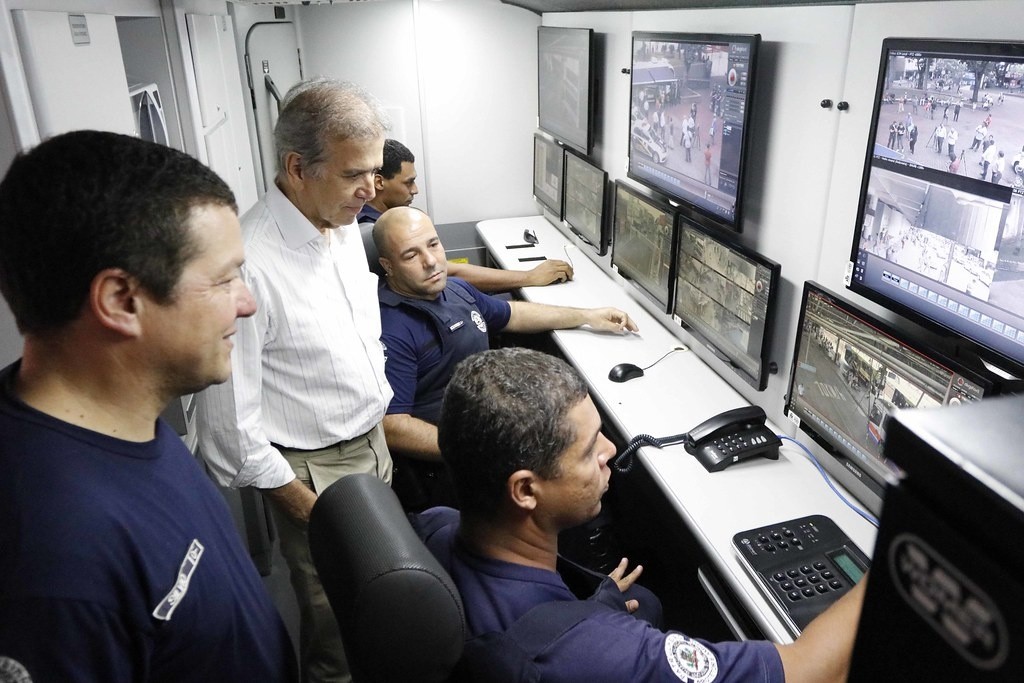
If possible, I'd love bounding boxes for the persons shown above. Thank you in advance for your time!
[639,83,722,185]
[0,129,300,683]
[372,207,639,570]
[882,91,1005,183]
[408,347,867,683]
[864,226,976,295]
[195,74,394,683]
[797,383,804,397]
[813,326,858,387]
[356,139,574,293]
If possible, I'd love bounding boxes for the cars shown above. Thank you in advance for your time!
[631,120,670,165]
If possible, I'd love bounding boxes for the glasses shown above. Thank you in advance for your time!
[524,229,538,243]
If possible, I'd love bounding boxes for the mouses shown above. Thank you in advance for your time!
[609,363,644,382]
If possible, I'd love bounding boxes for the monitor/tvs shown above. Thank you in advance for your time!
[627,31,762,232]
[537,26,595,155]
[563,150,608,257]
[670,213,782,391]
[783,280,1004,500]
[844,36,1024,379]
[532,133,566,222]
[609,179,680,314]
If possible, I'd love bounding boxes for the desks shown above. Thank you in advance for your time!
[476,215,879,646]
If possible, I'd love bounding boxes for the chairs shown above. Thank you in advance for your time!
[310,473,464,683]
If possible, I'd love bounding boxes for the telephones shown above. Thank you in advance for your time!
[684,405,784,473]
